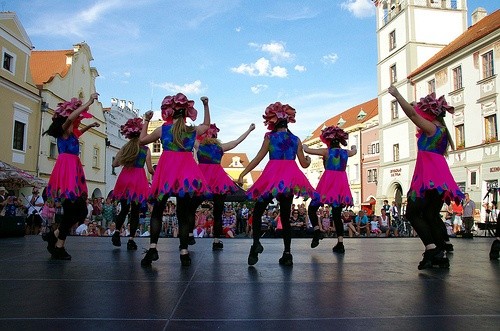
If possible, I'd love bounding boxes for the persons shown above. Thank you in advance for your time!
[302,125,357,253]
[71,198,417,239]
[111,118,155,250]
[42,92,101,260]
[388,85,468,270]
[462,193,475,234]
[444,201,463,236]
[0,186,64,235]
[238,102,311,267]
[482,200,500,223]
[188,122,255,250]
[489,211,500,261]
[138,92,211,266]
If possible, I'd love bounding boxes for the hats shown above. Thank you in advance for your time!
[0,187,6,191]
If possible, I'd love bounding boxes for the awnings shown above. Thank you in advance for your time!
[0,160,49,190]
[361,199,376,206]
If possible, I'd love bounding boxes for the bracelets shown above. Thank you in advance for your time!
[145,118,150,123]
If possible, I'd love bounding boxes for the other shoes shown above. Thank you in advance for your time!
[213,241,223,249]
[439,242,454,252]
[356,232,359,236]
[311,230,324,248]
[333,243,345,254]
[248,241,264,265]
[188,236,196,245]
[490,239,500,261]
[417,247,450,270]
[279,251,293,268]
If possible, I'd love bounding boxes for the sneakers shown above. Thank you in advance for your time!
[112,232,122,246]
[141,247,160,268]
[127,239,138,250]
[179,251,191,266]
[42,232,72,260]
